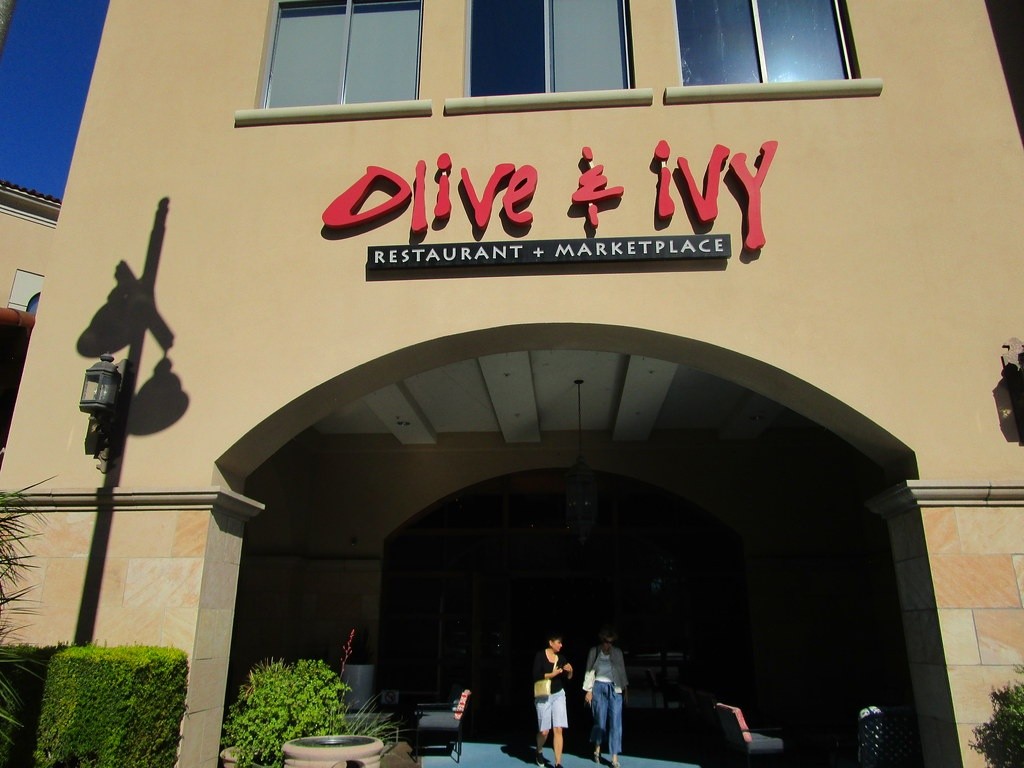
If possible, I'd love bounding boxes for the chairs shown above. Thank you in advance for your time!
[695,692,764,762]
[682,687,739,750]
[716,704,785,768]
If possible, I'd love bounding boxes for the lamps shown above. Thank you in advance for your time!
[78,353,121,475]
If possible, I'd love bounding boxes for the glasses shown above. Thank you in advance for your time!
[604,638,617,644]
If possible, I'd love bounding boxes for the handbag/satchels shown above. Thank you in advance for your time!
[534,679,551,698]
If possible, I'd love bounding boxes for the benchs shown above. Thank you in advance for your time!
[414,684,472,762]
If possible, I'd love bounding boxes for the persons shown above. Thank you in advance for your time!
[533,636,574,768]
[583,630,628,768]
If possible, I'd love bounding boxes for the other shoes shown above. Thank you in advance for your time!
[594,751,601,763]
[611,761,620,768]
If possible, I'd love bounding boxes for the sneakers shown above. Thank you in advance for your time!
[555,764,563,768]
[536,747,544,766]
